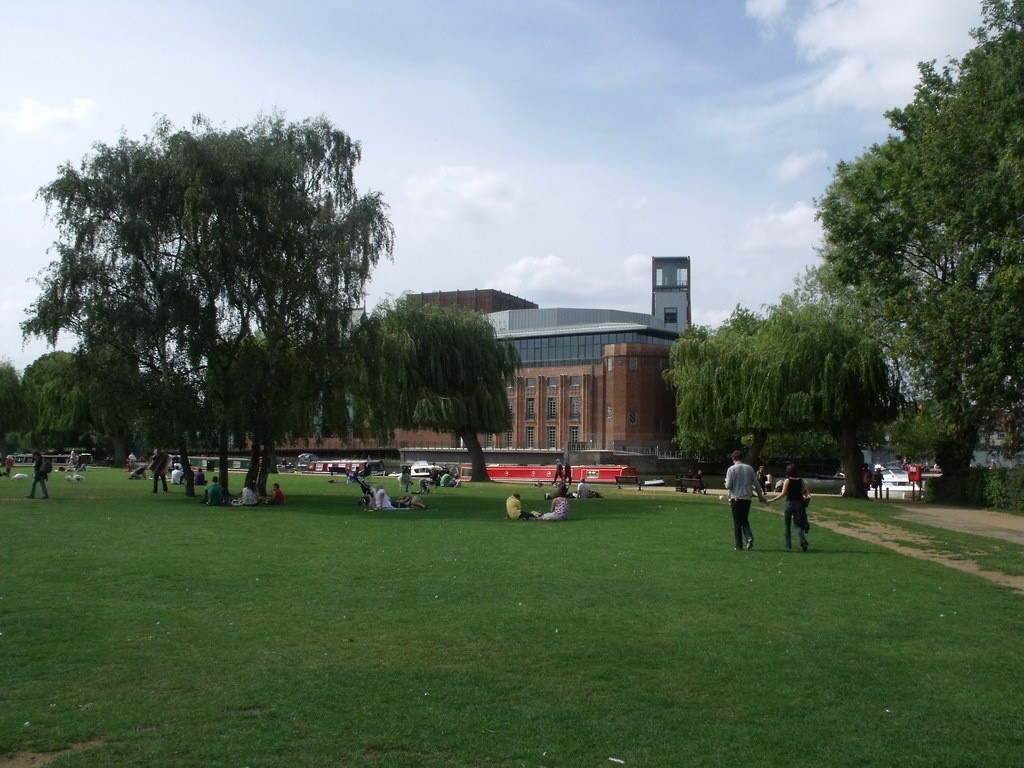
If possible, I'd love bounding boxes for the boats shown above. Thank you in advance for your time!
[167,455,298,474]
[840,464,926,501]
[454,462,639,485]
[7,452,92,466]
[295,459,389,476]
[388,460,442,476]
[641,479,665,486]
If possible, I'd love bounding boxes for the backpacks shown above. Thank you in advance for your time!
[39,458,51,478]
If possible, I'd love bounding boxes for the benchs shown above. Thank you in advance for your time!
[329,467,347,476]
[680,477,709,494]
[615,475,644,491]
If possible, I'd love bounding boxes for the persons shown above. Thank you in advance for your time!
[723,450,766,551]
[863,462,884,499]
[767,464,811,553]
[0,447,783,521]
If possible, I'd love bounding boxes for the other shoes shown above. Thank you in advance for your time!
[747,539,752,549]
[803,543,807,551]
